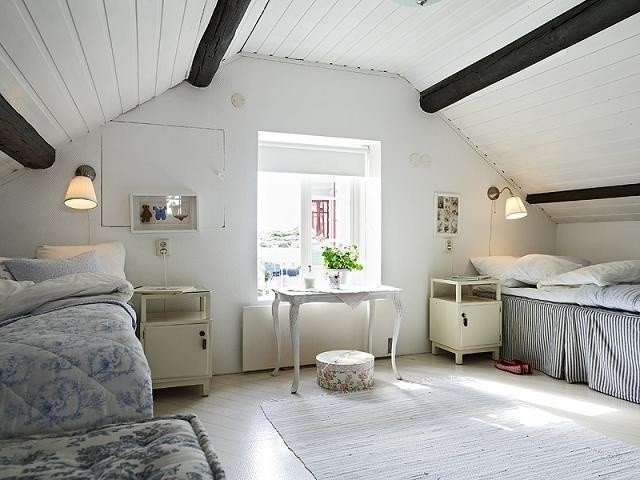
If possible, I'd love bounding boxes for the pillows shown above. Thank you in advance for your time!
[0,414,225,479]
[36,241,126,272]
[537,259,640,289]
[470,255,530,287]
[3,251,105,284]
[505,253,592,286]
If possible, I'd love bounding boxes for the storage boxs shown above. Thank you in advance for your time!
[315,350,375,392]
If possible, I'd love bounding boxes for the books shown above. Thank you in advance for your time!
[452,276,490,281]
[134,286,194,293]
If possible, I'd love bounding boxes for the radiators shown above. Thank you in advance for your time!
[242,299,392,373]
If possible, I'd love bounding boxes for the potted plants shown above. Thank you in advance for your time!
[321,244,364,289]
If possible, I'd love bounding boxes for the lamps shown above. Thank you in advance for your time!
[64,165,99,209]
[487,186,528,220]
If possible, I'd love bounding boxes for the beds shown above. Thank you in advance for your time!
[473,283,640,404]
[0,257,155,437]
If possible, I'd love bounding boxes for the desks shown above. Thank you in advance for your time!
[270,284,405,394]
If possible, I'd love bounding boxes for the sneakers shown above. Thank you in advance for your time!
[495,359,531,375]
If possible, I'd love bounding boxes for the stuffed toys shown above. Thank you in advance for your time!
[140,205,153,223]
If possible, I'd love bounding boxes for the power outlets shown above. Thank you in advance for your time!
[156,239,171,257]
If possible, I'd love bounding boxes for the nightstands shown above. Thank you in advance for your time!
[139,286,212,396]
[429,274,503,364]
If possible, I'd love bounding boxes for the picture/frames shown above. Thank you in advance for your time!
[434,192,461,237]
[129,193,199,232]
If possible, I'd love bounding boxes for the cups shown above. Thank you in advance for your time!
[304,278,315,290]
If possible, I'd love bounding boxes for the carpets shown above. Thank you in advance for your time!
[260,376,640,480]
